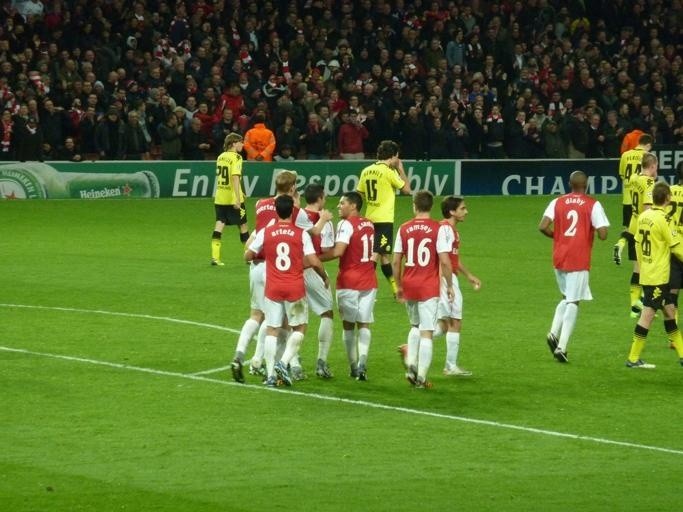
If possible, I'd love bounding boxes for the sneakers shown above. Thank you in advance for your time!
[612,243,622,266]
[228,343,476,390]
[211,258,225,267]
[629,302,657,319]
[624,358,657,370]
[544,332,568,362]
